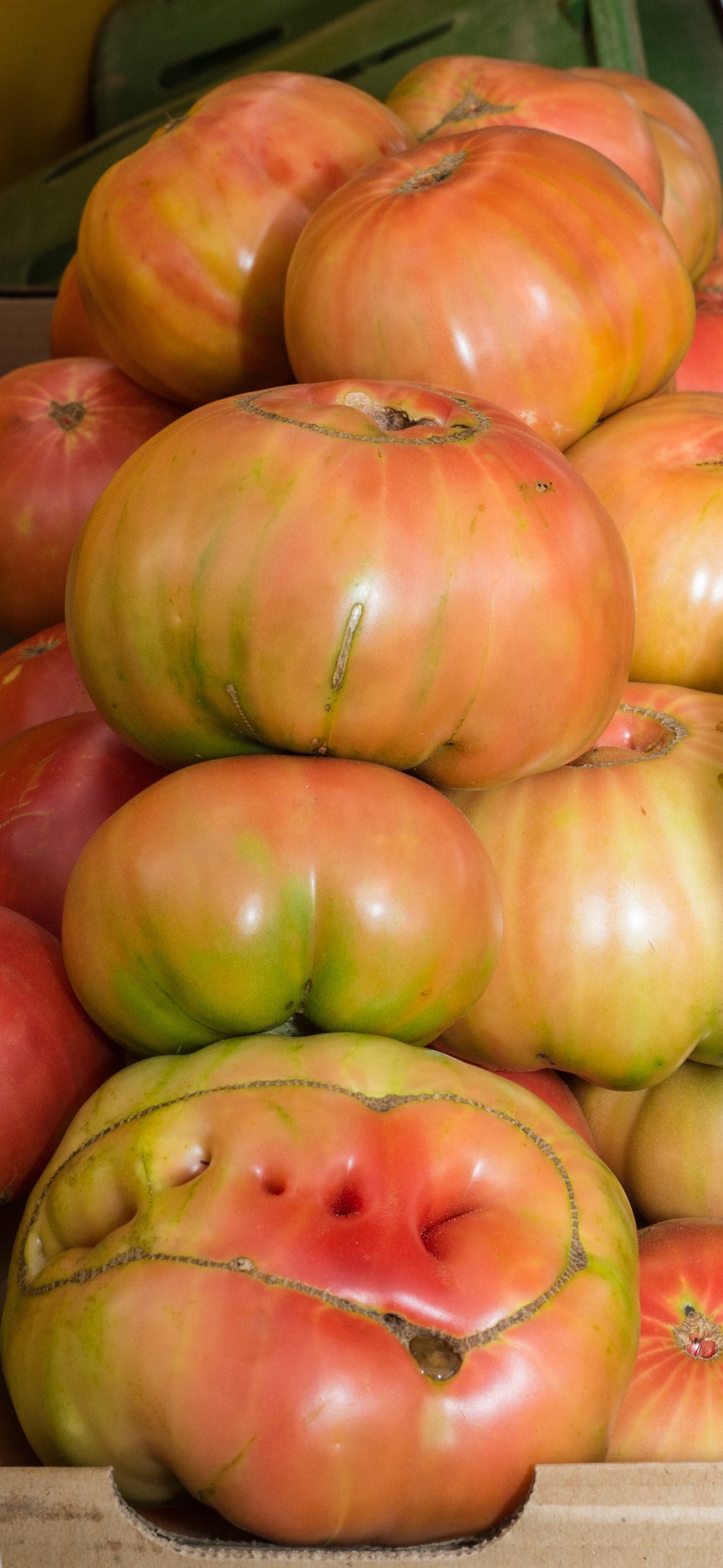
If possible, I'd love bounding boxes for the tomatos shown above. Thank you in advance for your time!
[0,54,722,1545]
